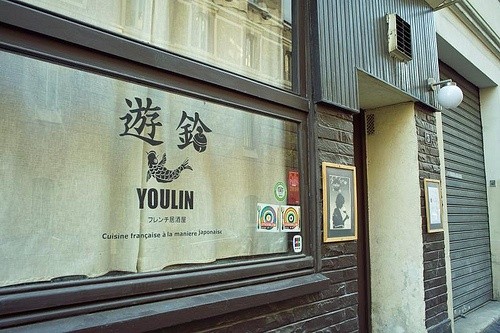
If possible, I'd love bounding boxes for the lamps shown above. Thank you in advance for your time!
[429,78,464,109]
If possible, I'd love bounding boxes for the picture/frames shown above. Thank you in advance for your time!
[424,177,444,233]
[321,162,358,243]
